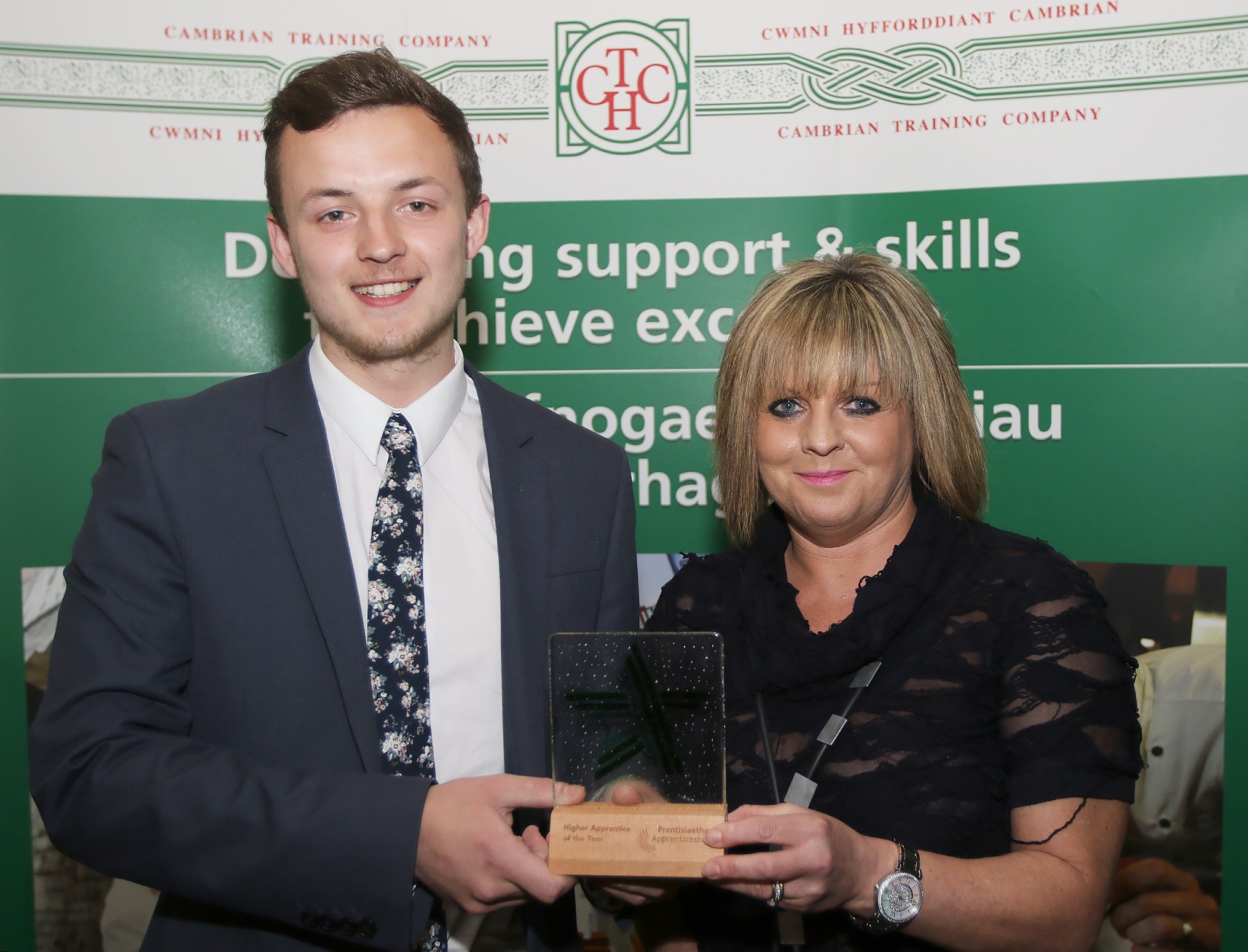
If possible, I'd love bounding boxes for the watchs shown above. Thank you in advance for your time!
[842,836,925,936]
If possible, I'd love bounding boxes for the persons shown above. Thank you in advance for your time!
[1106,850,1222,952]
[581,250,1152,952]
[29,52,643,952]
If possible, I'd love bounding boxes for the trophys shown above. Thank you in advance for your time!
[545,628,730,879]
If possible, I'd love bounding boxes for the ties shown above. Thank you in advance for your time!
[365,416,439,784]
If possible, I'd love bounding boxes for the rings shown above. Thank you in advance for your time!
[767,881,784,907]
[1183,920,1193,943]
